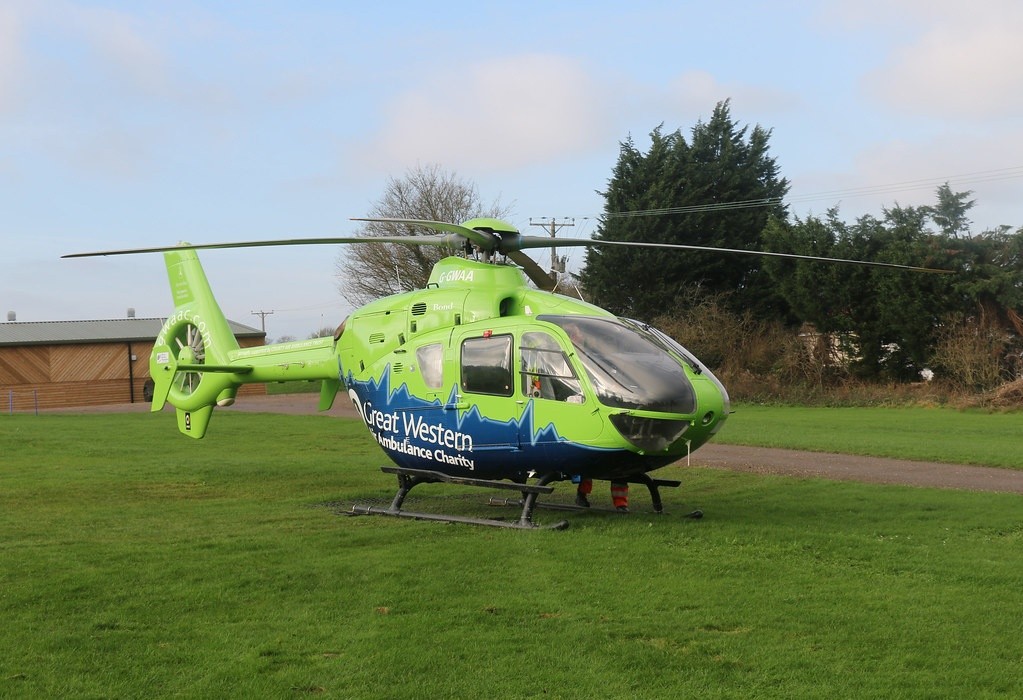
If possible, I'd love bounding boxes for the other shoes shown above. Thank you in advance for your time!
[617,505,629,512]
[574,493,590,508]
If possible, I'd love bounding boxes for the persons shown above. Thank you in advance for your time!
[575,476,629,515]
[561,322,583,345]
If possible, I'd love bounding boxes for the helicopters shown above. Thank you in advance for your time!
[60,167,956,532]
